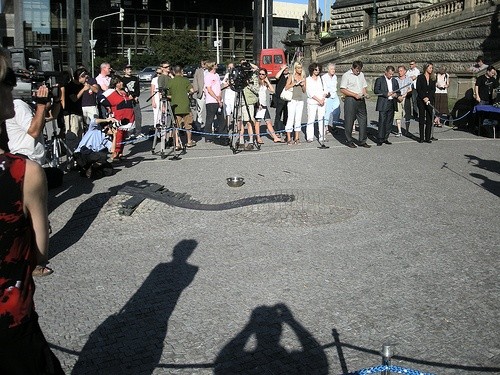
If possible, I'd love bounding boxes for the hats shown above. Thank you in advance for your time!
[74,69,87,80]
[124,64,133,70]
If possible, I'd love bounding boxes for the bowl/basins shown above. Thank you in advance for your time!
[226,177,244,187]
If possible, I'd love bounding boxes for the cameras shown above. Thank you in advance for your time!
[441,87,445,90]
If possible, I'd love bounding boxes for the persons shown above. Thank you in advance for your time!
[54,59,140,180]
[374,61,403,146]
[150,60,341,149]
[395,60,450,138]
[0,44,65,375]
[467,55,500,133]
[340,61,372,148]
[417,63,436,143]
[5,84,55,235]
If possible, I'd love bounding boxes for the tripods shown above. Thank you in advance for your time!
[230,89,261,153]
[153,99,186,158]
[47,118,74,168]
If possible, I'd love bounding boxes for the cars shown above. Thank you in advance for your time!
[139,66,157,82]
[181,65,198,77]
[216,64,226,75]
[114,64,138,78]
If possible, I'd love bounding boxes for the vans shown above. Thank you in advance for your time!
[259,48,286,82]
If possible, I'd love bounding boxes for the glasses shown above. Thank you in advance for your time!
[260,73,266,75]
[156,72,162,75]
[409,64,414,65]
[314,69,320,71]
[163,66,170,68]
[81,72,87,78]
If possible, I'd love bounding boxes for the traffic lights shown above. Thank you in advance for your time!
[119,7,125,21]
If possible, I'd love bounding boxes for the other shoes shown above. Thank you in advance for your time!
[405,132,413,137]
[345,142,356,148]
[384,139,392,145]
[419,139,423,143]
[425,140,431,143]
[111,155,119,161]
[258,138,265,143]
[175,145,180,151]
[359,142,367,147]
[274,128,340,145]
[395,133,402,136]
[119,153,123,159]
[31,266,53,276]
[187,142,196,148]
[377,139,382,146]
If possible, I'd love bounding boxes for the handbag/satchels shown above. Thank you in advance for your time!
[280,75,293,101]
[256,109,266,118]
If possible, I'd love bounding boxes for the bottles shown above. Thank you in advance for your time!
[218,106,221,115]
[84,82,88,93]
